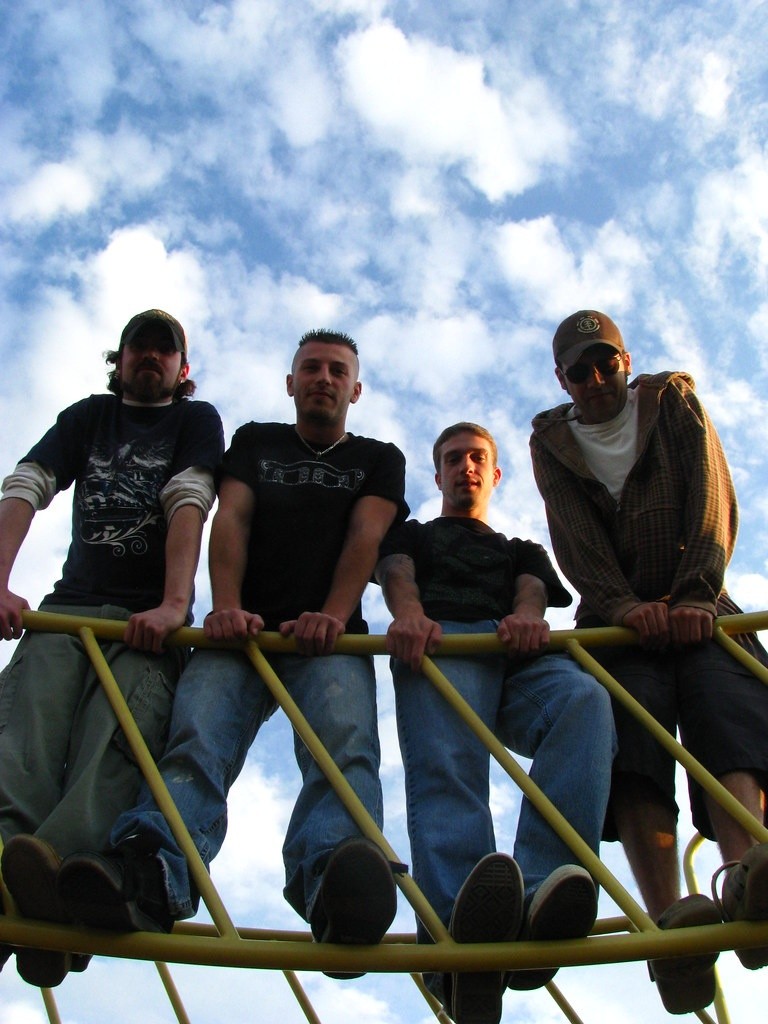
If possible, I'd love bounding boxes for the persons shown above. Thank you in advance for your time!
[370,424,612,1024]
[0,310,224,988]
[529,310,768,1014]
[52,331,406,980]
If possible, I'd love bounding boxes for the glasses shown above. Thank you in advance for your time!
[566,352,621,384]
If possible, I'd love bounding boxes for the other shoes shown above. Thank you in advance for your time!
[311,835,397,981]
[52,848,178,936]
[442,852,525,1024]
[647,893,723,1015]
[1,833,72,991]
[505,864,599,991]
[712,841,768,970]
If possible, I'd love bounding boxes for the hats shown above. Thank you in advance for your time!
[551,310,624,376]
[119,309,186,359]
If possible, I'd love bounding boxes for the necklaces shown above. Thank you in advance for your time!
[297,431,348,461]
